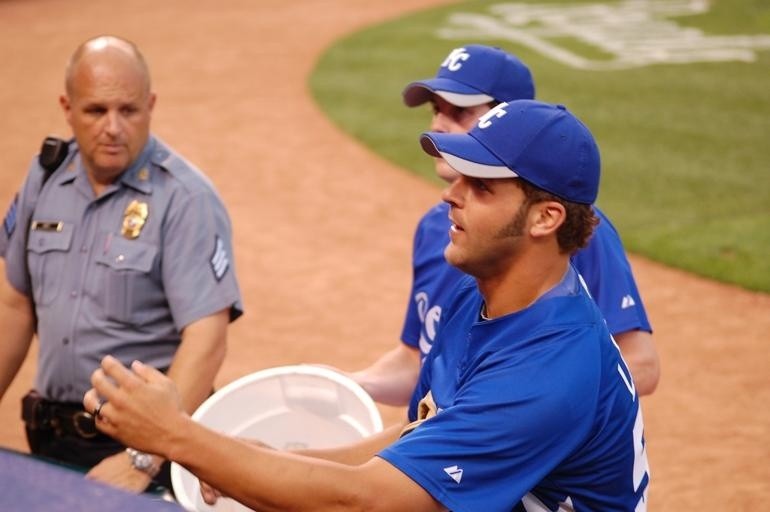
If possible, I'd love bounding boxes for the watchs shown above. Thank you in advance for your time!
[127,447,160,477]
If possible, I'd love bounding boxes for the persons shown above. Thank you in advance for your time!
[0,35,244,504]
[84,98,648,511]
[303,42,661,408]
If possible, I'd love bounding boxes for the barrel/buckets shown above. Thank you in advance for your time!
[170,366,381,511]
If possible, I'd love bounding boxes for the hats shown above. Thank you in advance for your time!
[403,43,602,204]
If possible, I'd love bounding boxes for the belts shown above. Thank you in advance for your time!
[21,394,110,439]
